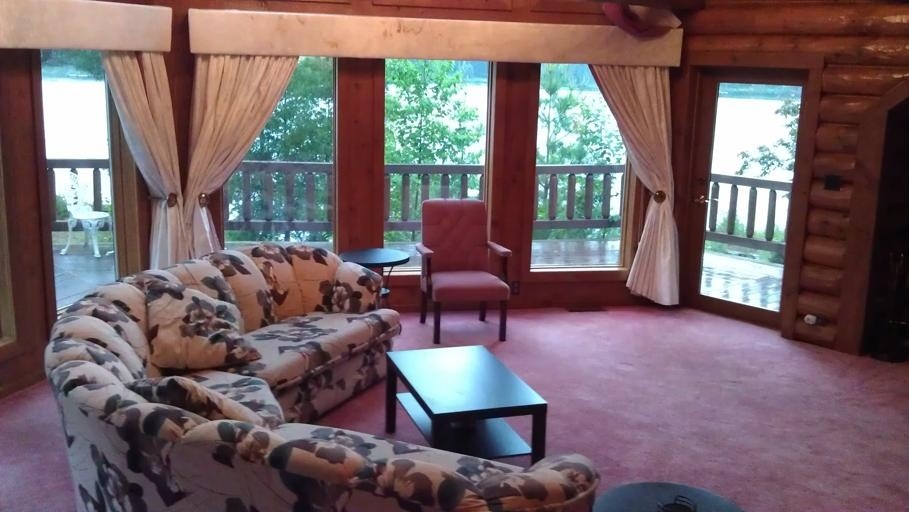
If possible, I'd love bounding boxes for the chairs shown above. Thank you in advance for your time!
[415,196,515,345]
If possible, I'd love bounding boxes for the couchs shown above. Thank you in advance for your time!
[40,244,601,511]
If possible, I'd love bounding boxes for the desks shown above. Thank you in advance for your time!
[338,248,411,310]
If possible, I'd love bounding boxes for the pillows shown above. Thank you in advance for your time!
[115,280,274,436]
